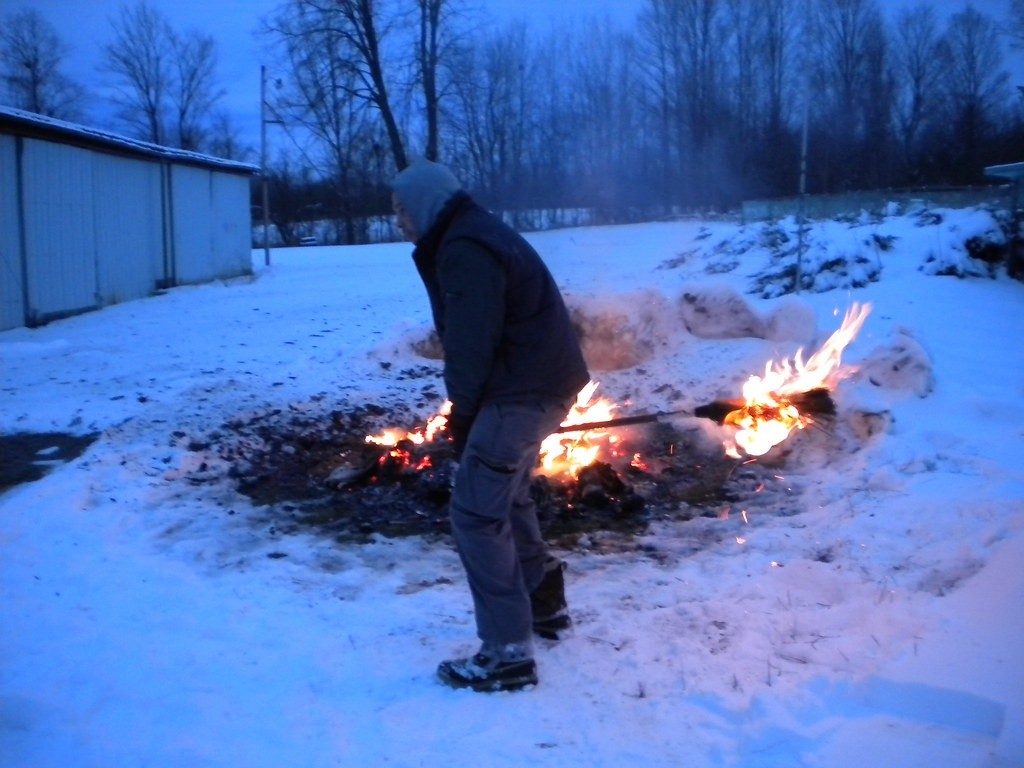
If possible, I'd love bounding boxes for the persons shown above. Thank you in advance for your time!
[386,158,592,690]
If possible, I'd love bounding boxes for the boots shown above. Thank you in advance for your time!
[436,641,539,692]
[532,556,574,639]
